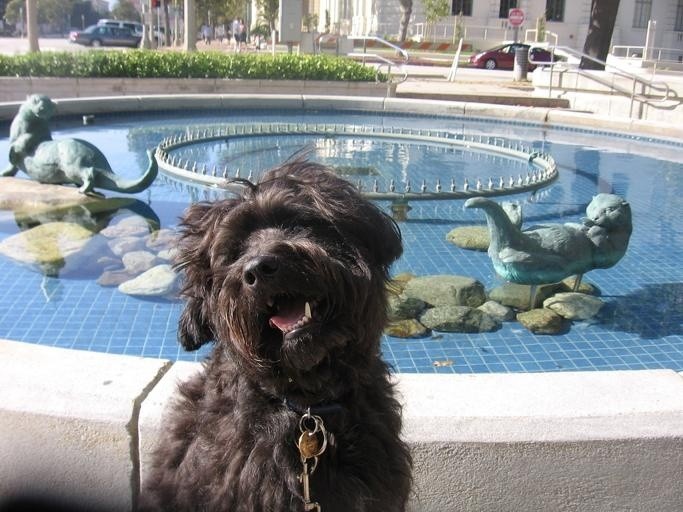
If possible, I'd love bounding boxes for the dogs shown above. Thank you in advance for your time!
[135,149,415,510]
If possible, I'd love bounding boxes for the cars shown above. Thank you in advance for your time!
[471,43,567,71]
[70,25,140,48]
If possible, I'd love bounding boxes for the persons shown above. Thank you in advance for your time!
[201,16,248,53]
[588,18,601,57]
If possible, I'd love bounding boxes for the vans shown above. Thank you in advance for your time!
[99,19,165,45]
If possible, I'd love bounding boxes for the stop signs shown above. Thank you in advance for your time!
[509,9,524,26]
